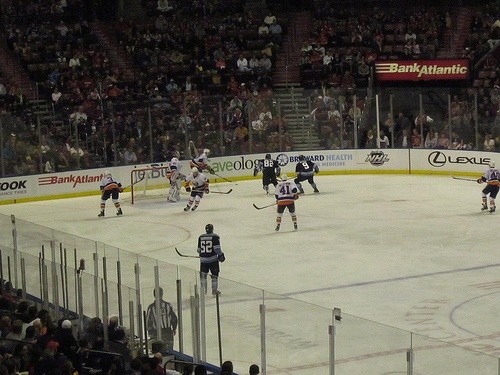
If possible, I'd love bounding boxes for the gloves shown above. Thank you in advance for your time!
[185,185,191,192]
[218,253,225,262]
[209,168,215,175]
[478,178,483,184]
[205,187,209,194]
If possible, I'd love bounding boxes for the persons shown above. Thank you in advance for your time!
[147,287,177,351]
[275,173,299,232]
[298,0,500,153]
[167,150,185,203]
[476,162,500,213]
[255,154,280,196]
[249,364,259,375]
[184,168,209,211]
[97,171,123,217]
[294,155,319,194]
[0,0,292,178]
[197,224,225,296]
[193,148,214,175]
[221,361,234,375]
[0,278,180,375]
[194,365,207,375]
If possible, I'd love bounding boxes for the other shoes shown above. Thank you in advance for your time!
[213,290,221,296]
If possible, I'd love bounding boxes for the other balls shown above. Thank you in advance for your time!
[235,184,238,185]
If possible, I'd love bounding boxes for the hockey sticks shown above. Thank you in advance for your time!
[253,203,276,210]
[175,247,199,258]
[452,175,477,182]
[123,173,145,190]
[276,176,298,182]
[188,189,232,194]
[213,172,232,182]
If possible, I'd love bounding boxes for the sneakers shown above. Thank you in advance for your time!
[116,208,122,216]
[298,191,305,195]
[264,190,269,195]
[275,225,280,232]
[170,196,177,202]
[184,205,190,211]
[313,188,319,193]
[489,206,496,212]
[481,203,488,211]
[294,222,298,230]
[98,210,104,217]
[191,205,198,211]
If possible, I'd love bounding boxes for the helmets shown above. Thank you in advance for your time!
[266,154,271,160]
[281,174,288,181]
[488,162,495,168]
[204,149,210,156]
[205,224,214,232]
[173,151,180,158]
[104,169,112,177]
[192,167,198,174]
[299,155,304,161]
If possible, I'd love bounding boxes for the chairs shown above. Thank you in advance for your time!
[324,22,404,61]
[23,52,61,76]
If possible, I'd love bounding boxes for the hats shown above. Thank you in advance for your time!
[47,341,59,351]
[62,319,71,329]
